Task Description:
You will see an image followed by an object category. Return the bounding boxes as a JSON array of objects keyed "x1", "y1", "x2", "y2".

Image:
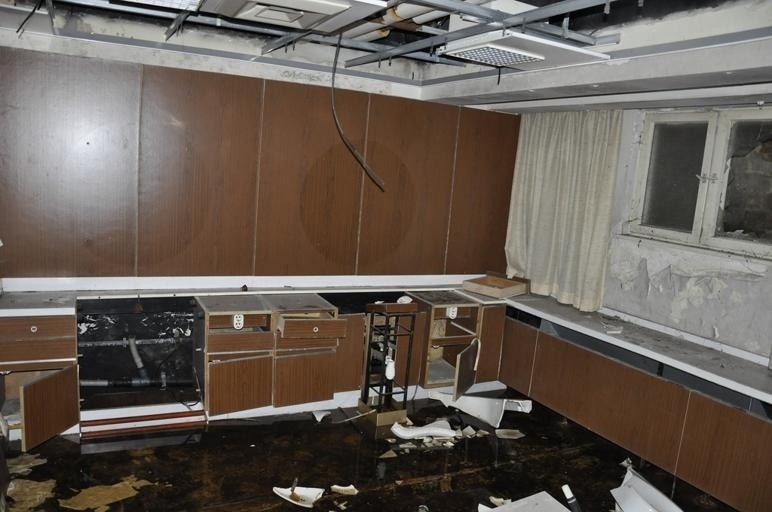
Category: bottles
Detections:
[{"x1": 385, "y1": 355, "x2": 395, "y2": 380}]
